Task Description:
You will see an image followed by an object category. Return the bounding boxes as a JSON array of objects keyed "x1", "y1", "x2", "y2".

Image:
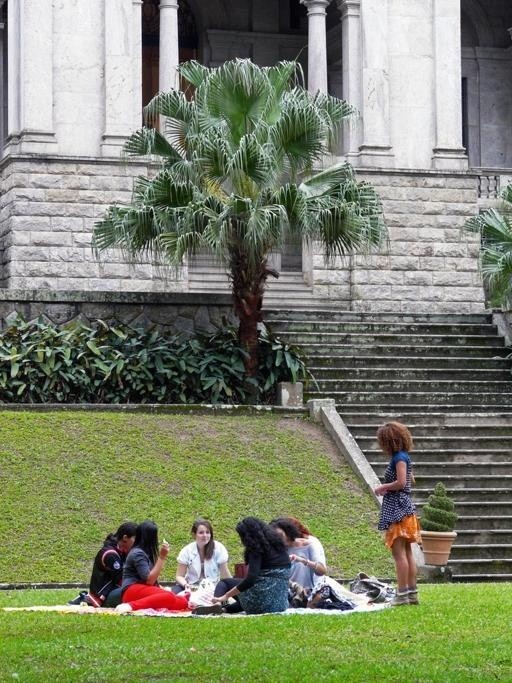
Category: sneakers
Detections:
[
  {"x1": 192, "y1": 604, "x2": 223, "y2": 616},
  {"x1": 387, "y1": 592, "x2": 409, "y2": 607},
  {"x1": 409, "y1": 590, "x2": 419, "y2": 605}
]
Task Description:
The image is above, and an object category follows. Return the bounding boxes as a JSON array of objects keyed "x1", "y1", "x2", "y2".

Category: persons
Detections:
[
  {"x1": 374, "y1": 420, "x2": 422, "y2": 606},
  {"x1": 191, "y1": 516, "x2": 291, "y2": 615},
  {"x1": 113, "y1": 519, "x2": 189, "y2": 614},
  {"x1": 86, "y1": 521, "x2": 139, "y2": 608},
  {"x1": 172, "y1": 519, "x2": 232, "y2": 593},
  {"x1": 269, "y1": 518, "x2": 327, "y2": 608}
]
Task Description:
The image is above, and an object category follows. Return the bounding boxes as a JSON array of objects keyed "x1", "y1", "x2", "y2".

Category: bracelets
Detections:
[{"x1": 313, "y1": 561, "x2": 319, "y2": 572}]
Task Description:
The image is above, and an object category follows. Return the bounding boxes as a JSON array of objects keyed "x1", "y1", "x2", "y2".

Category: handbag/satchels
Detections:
[{"x1": 350, "y1": 572, "x2": 395, "y2": 604}]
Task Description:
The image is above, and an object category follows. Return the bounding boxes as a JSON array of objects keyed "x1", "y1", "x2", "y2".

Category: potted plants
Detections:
[{"x1": 417, "y1": 480, "x2": 459, "y2": 566}]
